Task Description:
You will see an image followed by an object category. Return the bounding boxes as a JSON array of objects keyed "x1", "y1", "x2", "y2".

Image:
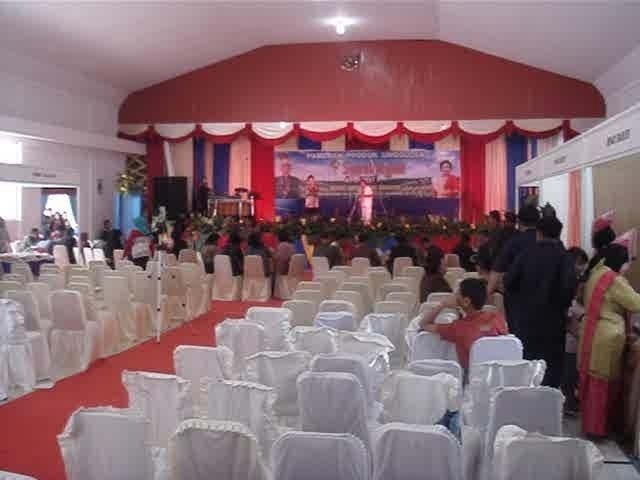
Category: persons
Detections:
[
  {"x1": 0, "y1": 207, "x2": 198, "y2": 273},
  {"x1": 357, "y1": 180, "x2": 374, "y2": 226},
  {"x1": 205, "y1": 213, "x2": 386, "y2": 277},
  {"x1": 304, "y1": 174, "x2": 320, "y2": 223},
  {"x1": 199, "y1": 175, "x2": 209, "y2": 217},
  {"x1": 432, "y1": 160, "x2": 460, "y2": 198},
  {"x1": 277, "y1": 161, "x2": 302, "y2": 199},
  {"x1": 390, "y1": 206, "x2": 639, "y2": 444}
]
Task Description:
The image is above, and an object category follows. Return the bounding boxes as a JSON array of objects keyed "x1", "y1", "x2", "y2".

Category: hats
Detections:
[{"x1": 132, "y1": 216, "x2": 147, "y2": 233}]
